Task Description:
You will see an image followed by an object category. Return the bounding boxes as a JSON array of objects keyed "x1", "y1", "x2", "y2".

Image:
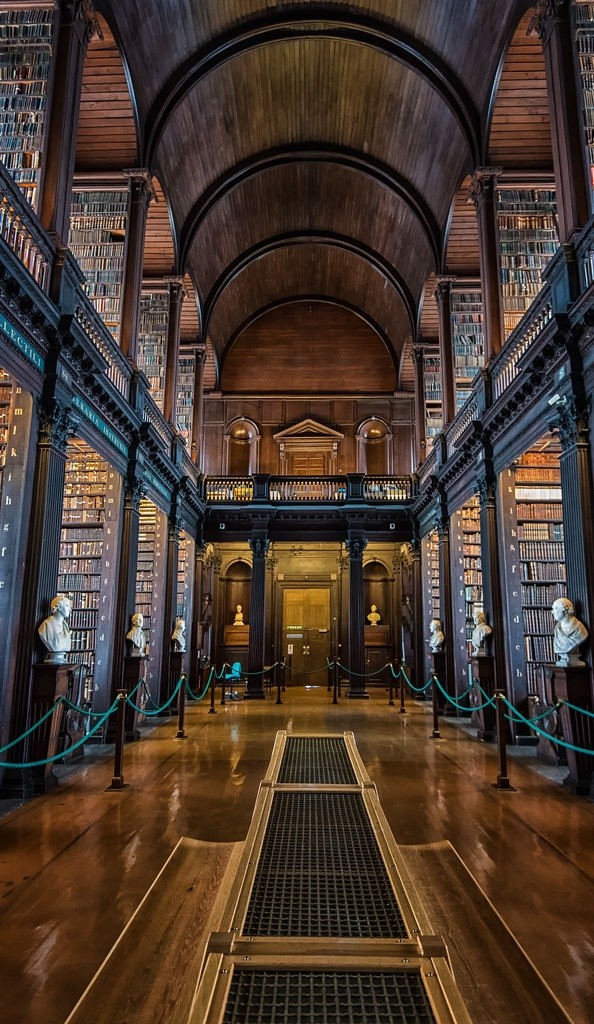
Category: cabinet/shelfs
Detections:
[
  {"x1": 410, "y1": 0, "x2": 594, "y2": 796},
  {"x1": 0, "y1": 0, "x2": 200, "y2": 744}
]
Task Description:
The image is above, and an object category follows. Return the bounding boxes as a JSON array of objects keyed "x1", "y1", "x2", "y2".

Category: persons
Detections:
[
  {"x1": 126, "y1": 614, "x2": 146, "y2": 653},
  {"x1": 171, "y1": 621, "x2": 187, "y2": 651},
  {"x1": 428, "y1": 620, "x2": 444, "y2": 646},
  {"x1": 470, "y1": 611, "x2": 491, "y2": 648},
  {"x1": 367, "y1": 605, "x2": 381, "y2": 621},
  {"x1": 550, "y1": 598, "x2": 588, "y2": 655},
  {"x1": 234, "y1": 604, "x2": 243, "y2": 620},
  {"x1": 38, "y1": 596, "x2": 74, "y2": 652}
]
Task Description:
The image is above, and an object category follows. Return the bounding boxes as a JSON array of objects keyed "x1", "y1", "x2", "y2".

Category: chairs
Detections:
[{"x1": 221, "y1": 661, "x2": 241, "y2": 701}]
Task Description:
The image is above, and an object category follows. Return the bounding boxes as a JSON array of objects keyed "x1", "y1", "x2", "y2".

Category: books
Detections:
[
  {"x1": 56, "y1": 448, "x2": 107, "y2": 705},
  {"x1": 137, "y1": 291, "x2": 168, "y2": 416},
  {"x1": 68, "y1": 190, "x2": 129, "y2": 347},
  {"x1": 498, "y1": 188, "x2": 559, "y2": 345},
  {"x1": 461, "y1": 497, "x2": 484, "y2": 650},
  {"x1": 0, "y1": 7, "x2": 54, "y2": 211},
  {"x1": 175, "y1": 357, "x2": 195, "y2": 456},
  {"x1": 513, "y1": 452, "x2": 567, "y2": 696},
  {"x1": 177, "y1": 529, "x2": 186, "y2": 618},
  {"x1": 134, "y1": 524, "x2": 155, "y2": 656},
  {"x1": 425, "y1": 358, "x2": 442, "y2": 400},
  {"x1": 0, "y1": 368, "x2": 14, "y2": 470},
  {"x1": 430, "y1": 527, "x2": 440, "y2": 619},
  {"x1": 451, "y1": 292, "x2": 483, "y2": 415},
  {"x1": 571, "y1": 0, "x2": 594, "y2": 168}
]
